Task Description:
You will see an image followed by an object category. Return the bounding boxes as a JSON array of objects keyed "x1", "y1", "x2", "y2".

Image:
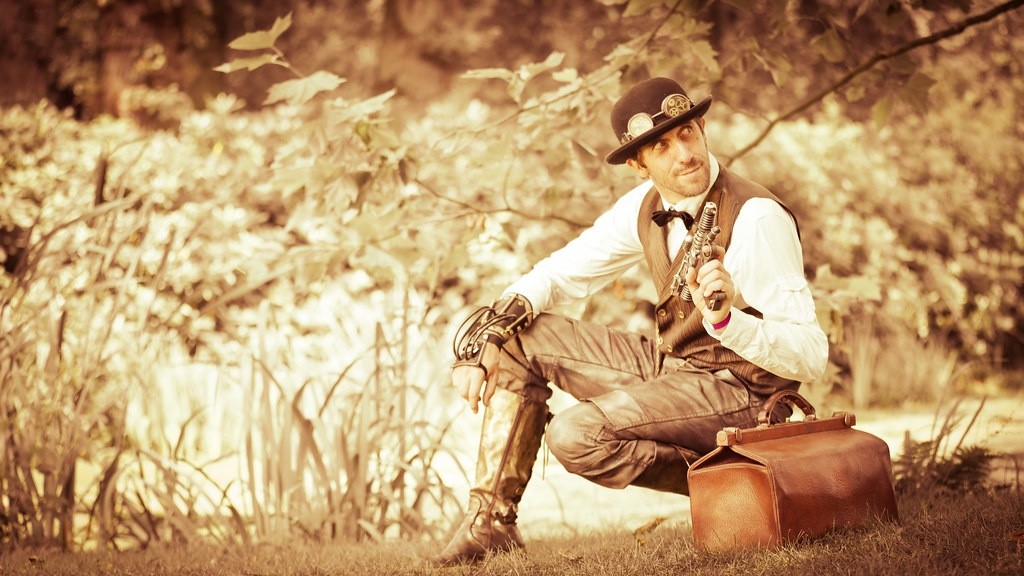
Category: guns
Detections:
[{"x1": 670, "y1": 201, "x2": 725, "y2": 312}]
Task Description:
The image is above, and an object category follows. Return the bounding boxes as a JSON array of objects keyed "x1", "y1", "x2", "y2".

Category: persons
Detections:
[{"x1": 404, "y1": 76, "x2": 830, "y2": 570}]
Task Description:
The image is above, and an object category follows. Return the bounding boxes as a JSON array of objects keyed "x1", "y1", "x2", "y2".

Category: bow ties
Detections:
[{"x1": 651, "y1": 209, "x2": 695, "y2": 231}]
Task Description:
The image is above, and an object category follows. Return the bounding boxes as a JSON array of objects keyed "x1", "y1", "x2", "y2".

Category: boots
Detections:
[
  {"x1": 629, "y1": 439, "x2": 704, "y2": 498},
  {"x1": 418, "y1": 384, "x2": 554, "y2": 568}
]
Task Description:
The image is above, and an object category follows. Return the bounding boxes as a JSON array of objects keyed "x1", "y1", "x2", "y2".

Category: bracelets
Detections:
[{"x1": 710, "y1": 311, "x2": 733, "y2": 331}]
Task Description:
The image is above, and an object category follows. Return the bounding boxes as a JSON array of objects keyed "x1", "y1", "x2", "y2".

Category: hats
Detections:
[{"x1": 605, "y1": 77, "x2": 712, "y2": 165}]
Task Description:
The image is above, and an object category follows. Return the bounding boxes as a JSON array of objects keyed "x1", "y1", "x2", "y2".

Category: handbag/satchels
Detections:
[{"x1": 687, "y1": 389, "x2": 898, "y2": 559}]
